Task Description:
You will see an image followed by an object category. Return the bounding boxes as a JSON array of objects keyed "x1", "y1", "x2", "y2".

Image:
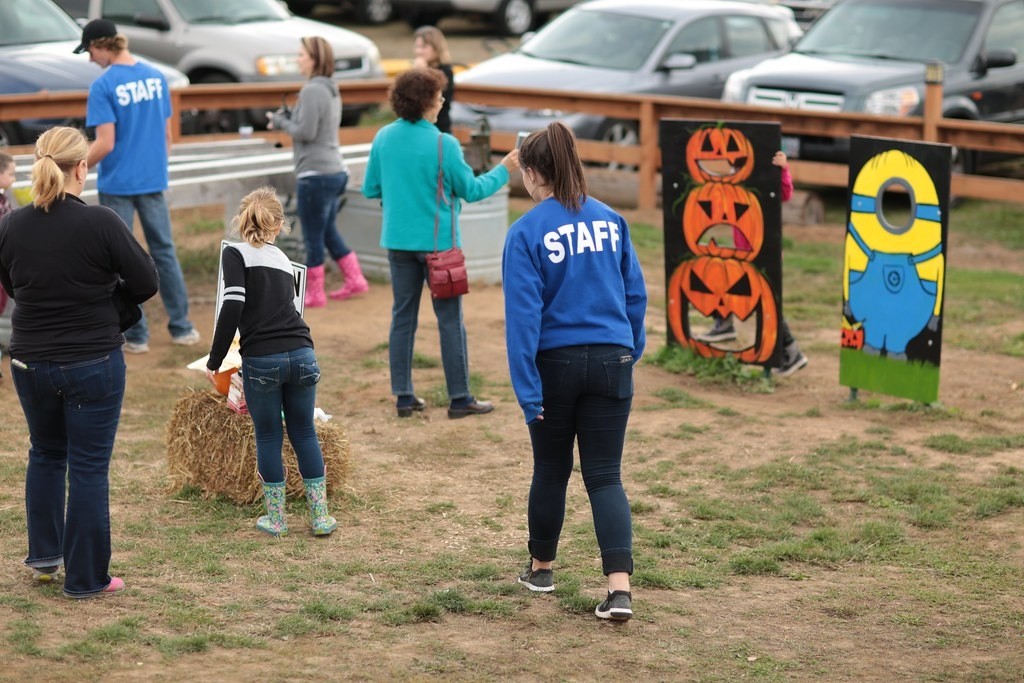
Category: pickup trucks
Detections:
[{"x1": 55, "y1": 0, "x2": 386, "y2": 135}]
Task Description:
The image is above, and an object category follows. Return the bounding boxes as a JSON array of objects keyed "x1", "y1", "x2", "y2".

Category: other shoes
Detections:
[
  {"x1": 448, "y1": 394, "x2": 495, "y2": 418},
  {"x1": 397, "y1": 392, "x2": 425, "y2": 416},
  {"x1": 63, "y1": 577, "x2": 125, "y2": 599},
  {"x1": 122, "y1": 340, "x2": 148, "y2": 354},
  {"x1": 33, "y1": 563, "x2": 58, "y2": 581},
  {"x1": 171, "y1": 328, "x2": 199, "y2": 346}
]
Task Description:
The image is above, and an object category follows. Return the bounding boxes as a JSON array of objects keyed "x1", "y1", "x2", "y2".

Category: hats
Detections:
[{"x1": 74, "y1": 17, "x2": 117, "y2": 55}]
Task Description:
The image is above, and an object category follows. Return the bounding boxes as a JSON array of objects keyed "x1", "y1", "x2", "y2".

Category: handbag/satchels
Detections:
[{"x1": 425, "y1": 247, "x2": 468, "y2": 298}]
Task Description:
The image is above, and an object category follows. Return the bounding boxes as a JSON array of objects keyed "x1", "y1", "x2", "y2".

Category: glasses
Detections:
[{"x1": 427, "y1": 96, "x2": 446, "y2": 104}]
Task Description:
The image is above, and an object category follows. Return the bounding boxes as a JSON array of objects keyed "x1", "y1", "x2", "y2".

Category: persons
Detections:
[
  {"x1": 0, "y1": 127, "x2": 159, "y2": 599},
  {"x1": 502, "y1": 121, "x2": 648, "y2": 621},
  {"x1": 413, "y1": 27, "x2": 454, "y2": 136},
  {"x1": 265, "y1": 36, "x2": 369, "y2": 308},
  {"x1": 72, "y1": 20, "x2": 201, "y2": 353},
  {"x1": 360, "y1": 70, "x2": 521, "y2": 420},
  {"x1": 0, "y1": 153, "x2": 16, "y2": 377},
  {"x1": 206, "y1": 186, "x2": 338, "y2": 537}
]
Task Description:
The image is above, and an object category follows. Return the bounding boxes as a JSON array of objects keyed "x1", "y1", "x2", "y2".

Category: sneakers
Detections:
[
  {"x1": 595, "y1": 589, "x2": 633, "y2": 622},
  {"x1": 776, "y1": 342, "x2": 808, "y2": 377},
  {"x1": 697, "y1": 324, "x2": 737, "y2": 342},
  {"x1": 519, "y1": 557, "x2": 556, "y2": 592}
]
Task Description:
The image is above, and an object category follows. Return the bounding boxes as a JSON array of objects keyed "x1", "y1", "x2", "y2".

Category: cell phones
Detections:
[{"x1": 516, "y1": 132, "x2": 531, "y2": 149}]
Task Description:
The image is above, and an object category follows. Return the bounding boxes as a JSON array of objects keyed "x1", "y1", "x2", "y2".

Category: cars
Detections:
[
  {"x1": 0, "y1": 0, "x2": 203, "y2": 148},
  {"x1": 280, "y1": 0, "x2": 542, "y2": 40},
  {"x1": 718, "y1": 0, "x2": 1024, "y2": 210},
  {"x1": 447, "y1": 0, "x2": 807, "y2": 175}
]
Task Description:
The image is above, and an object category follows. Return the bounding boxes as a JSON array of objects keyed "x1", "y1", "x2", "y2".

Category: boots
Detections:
[
  {"x1": 296, "y1": 465, "x2": 336, "y2": 535},
  {"x1": 255, "y1": 469, "x2": 289, "y2": 536},
  {"x1": 304, "y1": 266, "x2": 327, "y2": 306},
  {"x1": 329, "y1": 250, "x2": 368, "y2": 300}
]
